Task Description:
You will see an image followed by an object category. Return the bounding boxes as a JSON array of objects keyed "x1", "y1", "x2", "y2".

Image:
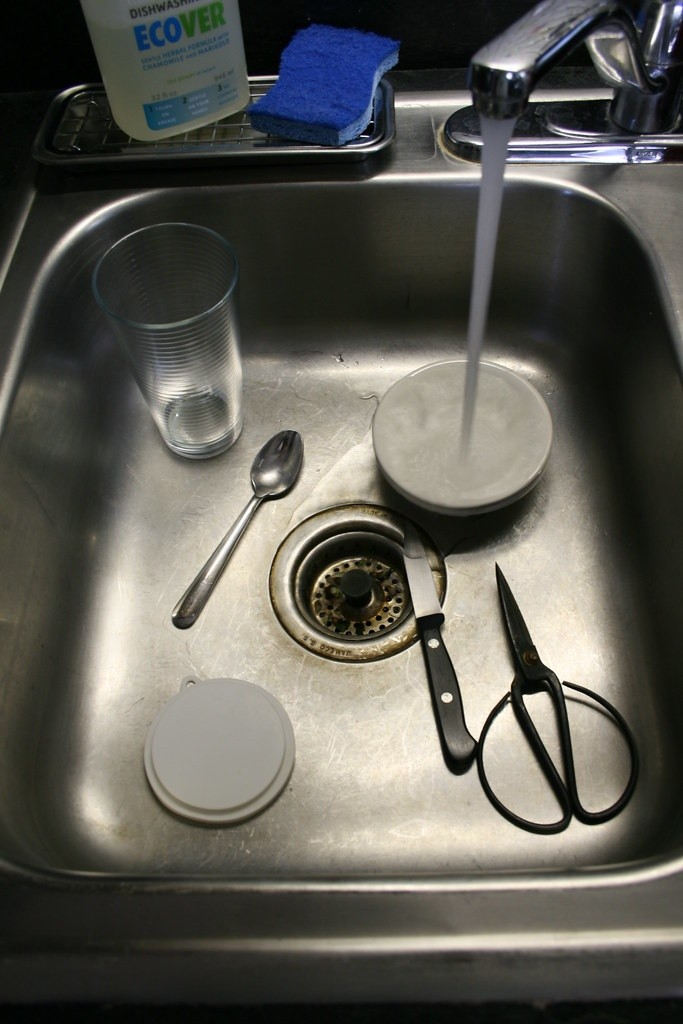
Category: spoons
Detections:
[{"x1": 171, "y1": 430, "x2": 304, "y2": 630}]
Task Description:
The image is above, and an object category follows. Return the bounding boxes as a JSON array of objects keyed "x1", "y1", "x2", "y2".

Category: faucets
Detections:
[{"x1": 467, "y1": 0, "x2": 683, "y2": 137}]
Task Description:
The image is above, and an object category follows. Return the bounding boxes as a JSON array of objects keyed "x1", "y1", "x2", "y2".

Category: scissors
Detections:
[{"x1": 477, "y1": 560, "x2": 641, "y2": 837}]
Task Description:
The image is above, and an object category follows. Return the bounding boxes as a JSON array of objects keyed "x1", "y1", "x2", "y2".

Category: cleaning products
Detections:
[{"x1": 78, "y1": 1, "x2": 253, "y2": 144}]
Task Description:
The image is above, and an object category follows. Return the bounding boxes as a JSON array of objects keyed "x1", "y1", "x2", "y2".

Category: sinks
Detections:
[{"x1": 0, "y1": 74, "x2": 683, "y2": 1009}]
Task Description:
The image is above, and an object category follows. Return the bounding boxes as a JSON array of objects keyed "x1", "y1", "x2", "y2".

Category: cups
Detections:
[{"x1": 91, "y1": 221, "x2": 244, "y2": 460}]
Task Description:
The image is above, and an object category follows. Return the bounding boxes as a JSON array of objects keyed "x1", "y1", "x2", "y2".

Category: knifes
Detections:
[{"x1": 402, "y1": 519, "x2": 478, "y2": 776}]
